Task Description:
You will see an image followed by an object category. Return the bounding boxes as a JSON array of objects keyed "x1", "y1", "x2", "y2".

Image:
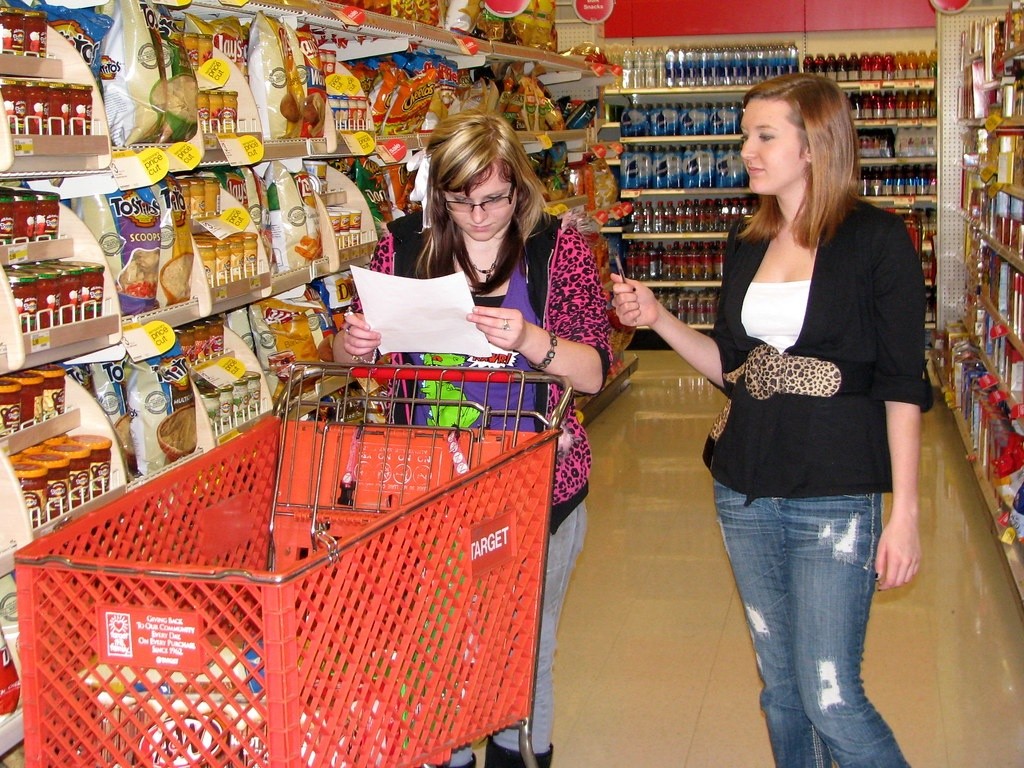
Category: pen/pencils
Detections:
[{"x1": 613, "y1": 250, "x2": 628, "y2": 285}]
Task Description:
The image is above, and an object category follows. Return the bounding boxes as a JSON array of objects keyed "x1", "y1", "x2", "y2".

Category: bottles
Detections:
[
  {"x1": 604, "y1": 41, "x2": 801, "y2": 331},
  {"x1": 803, "y1": 49, "x2": 940, "y2": 199}
]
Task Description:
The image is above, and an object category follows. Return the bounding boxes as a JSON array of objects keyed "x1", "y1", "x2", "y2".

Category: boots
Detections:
[{"x1": 484, "y1": 736, "x2": 554, "y2": 768}]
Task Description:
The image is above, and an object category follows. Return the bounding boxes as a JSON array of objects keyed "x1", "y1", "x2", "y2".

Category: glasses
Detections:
[{"x1": 442, "y1": 182, "x2": 516, "y2": 213}]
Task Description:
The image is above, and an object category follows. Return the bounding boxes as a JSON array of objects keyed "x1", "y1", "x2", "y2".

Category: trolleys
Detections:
[{"x1": 12, "y1": 357, "x2": 573, "y2": 768}]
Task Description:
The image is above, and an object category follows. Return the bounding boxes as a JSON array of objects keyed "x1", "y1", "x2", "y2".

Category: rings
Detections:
[
  {"x1": 346, "y1": 326, "x2": 351, "y2": 334},
  {"x1": 503, "y1": 320, "x2": 508, "y2": 330}
]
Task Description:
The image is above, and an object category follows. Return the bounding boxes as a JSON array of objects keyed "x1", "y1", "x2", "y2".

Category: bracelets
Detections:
[
  {"x1": 527, "y1": 332, "x2": 557, "y2": 370},
  {"x1": 356, "y1": 349, "x2": 377, "y2": 364}
]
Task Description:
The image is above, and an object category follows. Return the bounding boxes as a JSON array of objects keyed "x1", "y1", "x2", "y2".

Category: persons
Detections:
[
  {"x1": 334, "y1": 109, "x2": 613, "y2": 768},
  {"x1": 610, "y1": 73, "x2": 934, "y2": 768}
]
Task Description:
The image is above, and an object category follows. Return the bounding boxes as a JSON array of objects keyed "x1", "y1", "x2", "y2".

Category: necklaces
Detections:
[{"x1": 469, "y1": 256, "x2": 496, "y2": 281}]
